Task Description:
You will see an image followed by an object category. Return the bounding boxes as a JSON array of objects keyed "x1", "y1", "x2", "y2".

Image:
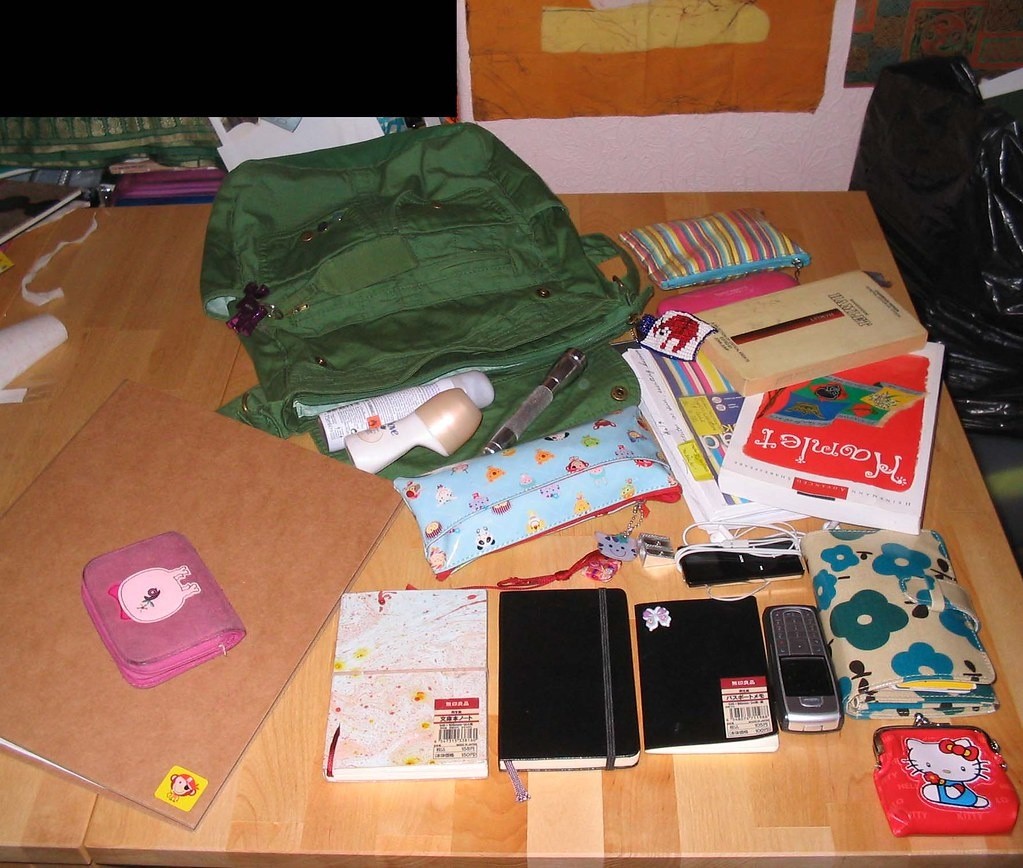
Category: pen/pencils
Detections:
[{"x1": 479, "y1": 347, "x2": 589, "y2": 457}]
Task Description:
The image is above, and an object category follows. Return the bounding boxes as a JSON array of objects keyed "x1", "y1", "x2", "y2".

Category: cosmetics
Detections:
[{"x1": 319, "y1": 370, "x2": 494, "y2": 474}]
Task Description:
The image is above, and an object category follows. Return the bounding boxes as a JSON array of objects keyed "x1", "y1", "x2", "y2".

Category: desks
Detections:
[{"x1": 0, "y1": 193, "x2": 1023, "y2": 868}]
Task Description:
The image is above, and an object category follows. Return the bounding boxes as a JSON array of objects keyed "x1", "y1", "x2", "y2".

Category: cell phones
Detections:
[
  {"x1": 677, "y1": 537, "x2": 804, "y2": 588},
  {"x1": 762, "y1": 605, "x2": 844, "y2": 735}
]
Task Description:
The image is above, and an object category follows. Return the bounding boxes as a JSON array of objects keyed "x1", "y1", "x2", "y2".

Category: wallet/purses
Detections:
[
  {"x1": 802, "y1": 528, "x2": 1001, "y2": 719},
  {"x1": 79, "y1": 530, "x2": 247, "y2": 688},
  {"x1": 873, "y1": 713, "x2": 1020, "y2": 837}
]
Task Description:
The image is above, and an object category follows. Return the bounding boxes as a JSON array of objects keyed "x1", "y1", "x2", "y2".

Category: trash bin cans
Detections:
[{"x1": 849, "y1": 53, "x2": 1023, "y2": 579}]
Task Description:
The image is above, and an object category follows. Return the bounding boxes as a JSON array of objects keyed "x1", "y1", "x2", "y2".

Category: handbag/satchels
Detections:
[
  {"x1": 620, "y1": 208, "x2": 811, "y2": 287},
  {"x1": 394, "y1": 405, "x2": 681, "y2": 580}
]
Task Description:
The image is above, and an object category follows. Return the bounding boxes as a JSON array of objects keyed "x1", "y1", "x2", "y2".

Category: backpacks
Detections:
[{"x1": 198, "y1": 121, "x2": 654, "y2": 481}]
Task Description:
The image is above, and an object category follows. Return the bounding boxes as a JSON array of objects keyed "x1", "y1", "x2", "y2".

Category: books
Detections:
[
  {"x1": 717, "y1": 342, "x2": 945, "y2": 535},
  {"x1": 498, "y1": 588, "x2": 641, "y2": 772},
  {"x1": 693, "y1": 270, "x2": 928, "y2": 397},
  {"x1": 0, "y1": 180, "x2": 81, "y2": 247},
  {"x1": 0, "y1": 380, "x2": 406, "y2": 831},
  {"x1": 635, "y1": 596, "x2": 781, "y2": 755},
  {"x1": 322, "y1": 588, "x2": 489, "y2": 783}
]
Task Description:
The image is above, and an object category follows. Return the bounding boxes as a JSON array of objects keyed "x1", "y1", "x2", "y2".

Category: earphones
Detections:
[{"x1": 710, "y1": 532, "x2": 726, "y2": 543}]
